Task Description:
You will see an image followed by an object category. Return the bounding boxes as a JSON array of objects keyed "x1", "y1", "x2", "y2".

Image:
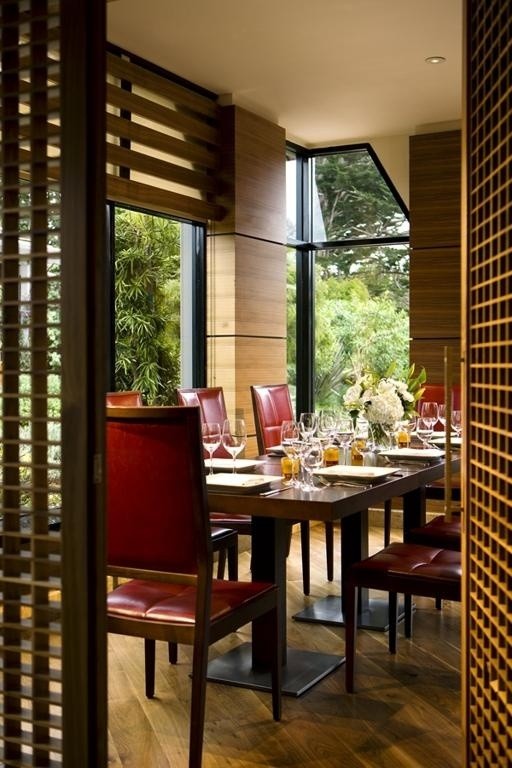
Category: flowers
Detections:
[{"x1": 343, "y1": 359, "x2": 426, "y2": 443}]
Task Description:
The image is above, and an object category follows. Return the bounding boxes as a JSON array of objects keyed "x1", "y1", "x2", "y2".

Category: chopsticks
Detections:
[{"x1": 204, "y1": 457, "x2": 268, "y2": 471}]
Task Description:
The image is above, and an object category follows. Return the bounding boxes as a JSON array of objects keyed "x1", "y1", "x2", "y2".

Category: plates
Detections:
[
  {"x1": 205, "y1": 471, "x2": 286, "y2": 493},
  {"x1": 377, "y1": 448, "x2": 446, "y2": 460},
  {"x1": 265, "y1": 444, "x2": 287, "y2": 454},
  {"x1": 407, "y1": 430, "x2": 456, "y2": 438},
  {"x1": 427, "y1": 436, "x2": 463, "y2": 444},
  {"x1": 313, "y1": 464, "x2": 402, "y2": 482}
]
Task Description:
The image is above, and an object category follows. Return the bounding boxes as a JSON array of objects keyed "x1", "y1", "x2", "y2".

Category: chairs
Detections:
[
  {"x1": 107, "y1": 406, "x2": 281, "y2": 767},
  {"x1": 346, "y1": 542, "x2": 461, "y2": 693},
  {"x1": 385, "y1": 384, "x2": 461, "y2": 547},
  {"x1": 107, "y1": 391, "x2": 238, "y2": 665},
  {"x1": 404, "y1": 516, "x2": 461, "y2": 637},
  {"x1": 251, "y1": 385, "x2": 334, "y2": 596},
  {"x1": 177, "y1": 388, "x2": 309, "y2": 595}
]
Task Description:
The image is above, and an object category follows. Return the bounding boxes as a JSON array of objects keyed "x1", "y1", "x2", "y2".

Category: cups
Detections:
[{"x1": 438, "y1": 404, "x2": 446, "y2": 425}]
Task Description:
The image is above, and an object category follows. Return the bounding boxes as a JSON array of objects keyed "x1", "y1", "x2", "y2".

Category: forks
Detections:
[{"x1": 317, "y1": 475, "x2": 368, "y2": 490}]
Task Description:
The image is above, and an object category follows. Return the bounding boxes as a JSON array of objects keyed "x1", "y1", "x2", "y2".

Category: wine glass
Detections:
[
  {"x1": 221, "y1": 418, "x2": 247, "y2": 474},
  {"x1": 416, "y1": 417, "x2": 434, "y2": 449},
  {"x1": 450, "y1": 410, "x2": 461, "y2": 438},
  {"x1": 421, "y1": 401, "x2": 439, "y2": 430},
  {"x1": 201, "y1": 422, "x2": 223, "y2": 475},
  {"x1": 281, "y1": 410, "x2": 369, "y2": 494}
]
